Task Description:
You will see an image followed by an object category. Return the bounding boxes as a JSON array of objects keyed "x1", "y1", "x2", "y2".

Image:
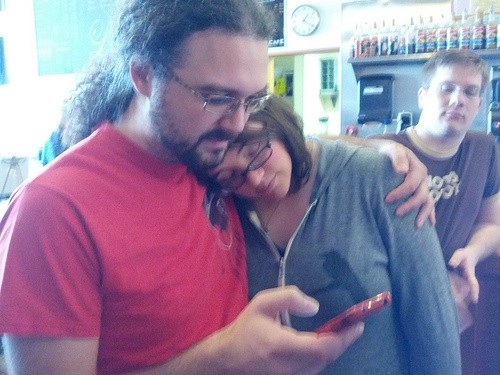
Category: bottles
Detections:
[
  {"x1": 316, "y1": 115, "x2": 329, "y2": 140},
  {"x1": 349, "y1": 0, "x2": 500, "y2": 57}
]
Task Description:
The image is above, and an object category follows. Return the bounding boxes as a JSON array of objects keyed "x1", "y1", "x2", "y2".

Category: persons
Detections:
[
  {"x1": 207, "y1": 92, "x2": 461, "y2": 375},
  {"x1": 370, "y1": 48, "x2": 500, "y2": 304},
  {"x1": 0, "y1": 20, "x2": 436, "y2": 375}
]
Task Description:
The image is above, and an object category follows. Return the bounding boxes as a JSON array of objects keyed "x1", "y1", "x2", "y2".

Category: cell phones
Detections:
[{"x1": 312, "y1": 291, "x2": 393, "y2": 335}]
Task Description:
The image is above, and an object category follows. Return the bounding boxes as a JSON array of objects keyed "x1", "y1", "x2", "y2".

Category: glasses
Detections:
[
  {"x1": 216, "y1": 122, "x2": 273, "y2": 197},
  {"x1": 162, "y1": 69, "x2": 272, "y2": 116}
]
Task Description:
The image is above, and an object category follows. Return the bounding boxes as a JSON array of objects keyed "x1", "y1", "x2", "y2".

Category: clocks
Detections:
[{"x1": 289, "y1": 4, "x2": 321, "y2": 36}]
